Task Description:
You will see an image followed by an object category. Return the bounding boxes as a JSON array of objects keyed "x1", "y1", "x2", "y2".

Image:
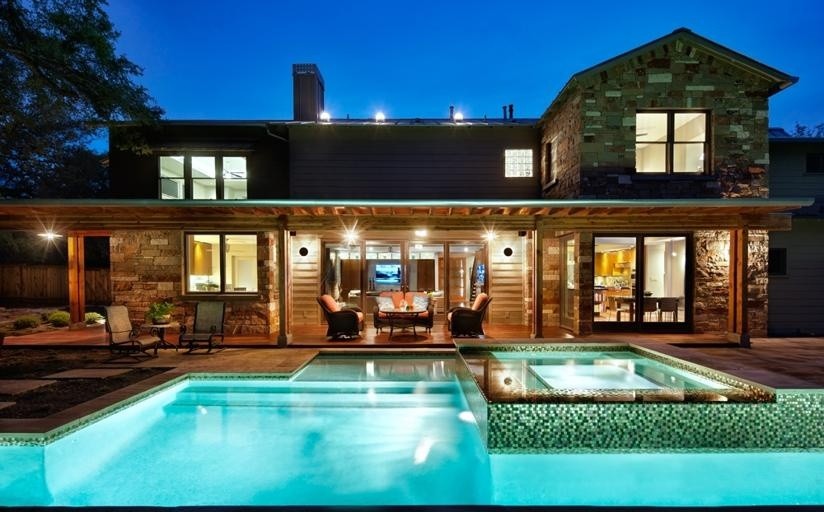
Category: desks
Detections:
[
  {"x1": 381, "y1": 308, "x2": 425, "y2": 340},
  {"x1": 141, "y1": 321, "x2": 181, "y2": 355}
]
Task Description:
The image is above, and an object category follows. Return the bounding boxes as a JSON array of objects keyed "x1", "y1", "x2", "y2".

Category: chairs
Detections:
[
  {"x1": 447, "y1": 292, "x2": 493, "y2": 338},
  {"x1": 103, "y1": 305, "x2": 161, "y2": 362},
  {"x1": 608, "y1": 296, "x2": 684, "y2": 322},
  {"x1": 179, "y1": 302, "x2": 227, "y2": 355},
  {"x1": 316, "y1": 294, "x2": 365, "y2": 339}
]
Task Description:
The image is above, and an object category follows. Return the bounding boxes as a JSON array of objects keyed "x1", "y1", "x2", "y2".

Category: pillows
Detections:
[
  {"x1": 376, "y1": 296, "x2": 394, "y2": 312},
  {"x1": 413, "y1": 296, "x2": 428, "y2": 311}
]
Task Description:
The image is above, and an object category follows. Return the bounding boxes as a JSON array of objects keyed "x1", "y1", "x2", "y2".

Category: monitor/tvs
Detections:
[
  {"x1": 469, "y1": 264, "x2": 486, "y2": 286},
  {"x1": 373, "y1": 262, "x2": 402, "y2": 286}
]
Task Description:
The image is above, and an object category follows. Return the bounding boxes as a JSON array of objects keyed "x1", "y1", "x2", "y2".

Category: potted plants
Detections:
[{"x1": 146, "y1": 301, "x2": 172, "y2": 323}]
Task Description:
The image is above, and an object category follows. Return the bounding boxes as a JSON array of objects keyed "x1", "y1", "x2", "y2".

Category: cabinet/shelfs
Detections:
[{"x1": 594, "y1": 252, "x2": 632, "y2": 287}]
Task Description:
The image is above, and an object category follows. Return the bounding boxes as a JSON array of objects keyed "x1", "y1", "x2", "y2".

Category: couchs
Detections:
[{"x1": 375, "y1": 291, "x2": 433, "y2": 333}]
